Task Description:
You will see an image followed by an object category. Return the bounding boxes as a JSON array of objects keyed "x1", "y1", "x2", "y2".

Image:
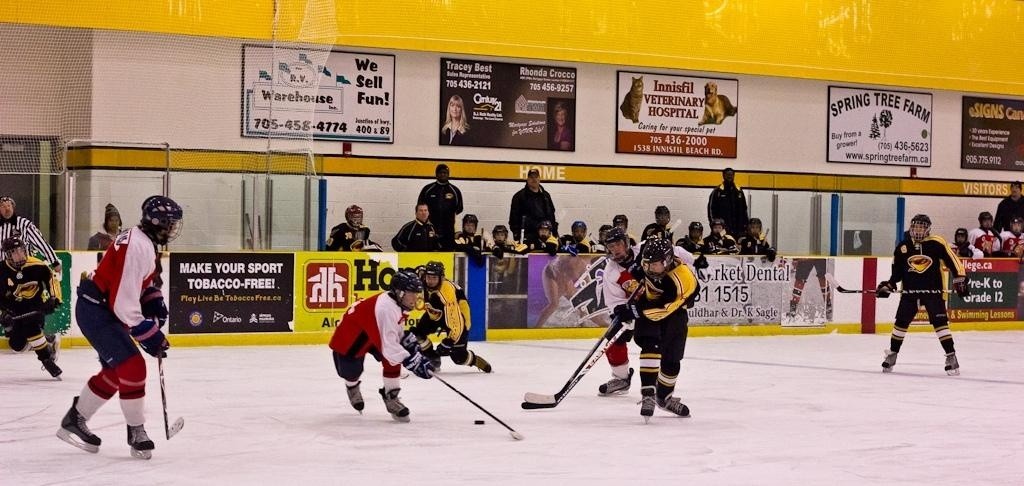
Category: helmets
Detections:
[
  {"x1": 345, "y1": 205, "x2": 363, "y2": 226},
  {"x1": 604, "y1": 226, "x2": 633, "y2": 253},
  {"x1": 1, "y1": 237, "x2": 28, "y2": 269},
  {"x1": 142, "y1": 195, "x2": 184, "y2": 244},
  {"x1": 389, "y1": 270, "x2": 423, "y2": 311},
  {"x1": 425, "y1": 260, "x2": 445, "y2": 288},
  {"x1": 613, "y1": 214, "x2": 628, "y2": 233},
  {"x1": 953, "y1": 228, "x2": 968, "y2": 247},
  {"x1": 600, "y1": 224, "x2": 612, "y2": 244},
  {"x1": 978, "y1": 212, "x2": 993, "y2": 229},
  {"x1": 1009, "y1": 216, "x2": 1024, "y2": 235},
  {"x1": 570, "y1": 221, "x2": 587, "y2": 240},
  {"x1": 640, "y1": 238, "x2": 675, "y2": 278},
  {"x1": 747, "y1": 218, "x2": 762, "y2": 238},
  {"x1": 655, "y1": 206, "x2": 670, "y2": 225},
  {"x1": 909, "y1": 214, "x2": 933, "y2": 238},
  {"x1": 493, "y1": 225, "x2": 508, "y2": 240},
  {"x1": 464, "y1": 214, "x2": 478, "y2": 236},
  {"x1": 688, "y1": 221, "x2": 703, "y2": 239},
  {"x1": 538, "y1": 220, "x2": 553, "y2": 239},
  {"x1": 710, "y1": 219, "x2": 724, "y2": 234}
]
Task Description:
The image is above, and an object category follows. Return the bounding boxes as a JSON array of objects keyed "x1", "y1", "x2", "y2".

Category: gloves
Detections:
[
  {"x1": 129, "y1": 320, "x2": 171, "y2": 359},
  {"x1": 38, "y1": 300, "x2": 57, "y2": 315},
  {"x1": 0, "y1": 308, "x2": 17, "y2": 330},
  {"x1": 141, "y1": 286, "x2": 168, "y2": 329},
  {"x1": 400, "y1": 331, "x2": 455, "y2": 379},
  {"x1": 953, "y1": 277, "x2": 971, "y2": 297},
  {"x1": 613, "y1": 305, "x2": 642, "y2": 321},
  {"x1": 876, "y1": 280, "x2": 897, "y2": 297}
]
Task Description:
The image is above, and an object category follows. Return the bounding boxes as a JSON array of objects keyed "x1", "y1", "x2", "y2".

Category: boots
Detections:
[
  {"x1": 944, "y1": 353, "x2": 959, "y2": 376},
  {"x1": 56, "y1": 395, "x2": 101, "y2": 453},
  {"x1": 38, "y1": 333, "x2": 63, "y2": 382},
  {"x1": 466, "y1": 353, "x2": 493, "y2": 373},
  {"x1": 656, "y1": 391, "x2": 691, "y2": 419},
  {"x1": 125, "y1": 423, "x2": 155, "y2": 460},
  {"x1": 882, "y1": 349, "x2": 897, "y2": 373},
  {"x1": 597, "y1": 367, "x2": 633, "y2": 397},
  {"x1": 637, "y1": 385, "x2": 658, "y2": 423},
  {"x1": 346, "y1": 381, "x2": 365, "y2": 415},
  {"x1": 379, "y1": 386, "x2": 409, "y2": 422}
]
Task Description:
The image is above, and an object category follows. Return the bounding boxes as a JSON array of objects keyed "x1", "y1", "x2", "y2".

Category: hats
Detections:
[
  {"x1": 1011, "y1": 180, "x2": 1021, "y2": 190},
  {"x1": 104, "y1": 203, "x2": 123, "y2": 231},
  {"x1": 528, "y1": 168, "x2": 540, "y2": 178},
  {"x1": 723, "y1": 167, "x2": 736, "y2": 174},
  {"x1": 434, "y1": 162, "x2": 450, "y2": 173}
]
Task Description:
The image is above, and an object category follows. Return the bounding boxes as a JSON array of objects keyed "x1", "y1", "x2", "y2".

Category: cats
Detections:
[{"x1": 619, "y1": 75, "x2": 644, "y2": 123}]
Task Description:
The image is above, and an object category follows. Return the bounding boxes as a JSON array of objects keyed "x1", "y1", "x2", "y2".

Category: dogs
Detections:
[{"x1": 697, "y1": 82, "x2": 736, "y2": 126}]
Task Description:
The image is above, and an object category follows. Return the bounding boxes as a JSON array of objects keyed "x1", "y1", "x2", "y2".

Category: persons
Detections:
[
  {"x1": 413, "y1": 164, "x2": 463, "y2": 246},
  {"x1": 998, "y1": 212, "x2": 1023, "y2": 258},
  {"x1": 994, "y1": 180, "x2": 1023, "y2": 233},
  {"x1": 55, "y1": 194, "x2": 183, "y2": 461},
  {"x1": 596, "y1": 227, "x2": 710, "y2": 396},
  {"x1": 613, "y1": 236, "x2": 691, "y2": 425},
  {"x1": 88, "y1": 203, "x2": 125, "y2": 250},
  {"x1": 967, "y1": 211, "x2": 1002, "y2": 257},
  {"x1": 595, "y1": 206, "x2": 776, "y2": 262},
  {"x1": 453, "y1": 214, "x2": 597, "y2": 259},
  {"x1": 0, "y1": 239, "x2": 65, "y2": 384},
  {"x1": 390, "y1": 199, "x2": 445, "y2": 251},
  {"x1": 438, "y1": 94, "x2": 476, "y2": 146},
  {"x1": 329, "y1": 270, "x2": 435, "y2": 422},
  {"x1": 947, "y1": 226, "x2": 983, "y2": 259},
  {"x1": 0, "y1": 197, "x2": 63, "y2": 275},
  {"x1": 708, "y1": 168, "x2": 748, "y2": 233},
  {"x1": 325, "y1": 204, "x2": 381, "y2": 250},
  {"x1": 547, "y1": 101, "x2": 574, "y2": 150},
  {"x1": 507, "y1": 169, "x2": 556, "y2": 243},
  {"x1": 411, "y1": 259, "x2": 496, "y2": 375},
  {"x1": 873, "y1": 213, "x2": 967, "y2": 375}
]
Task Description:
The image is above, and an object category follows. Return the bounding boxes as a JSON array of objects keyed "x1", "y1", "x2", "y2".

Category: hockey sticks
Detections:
[
  {"x1": 824, "y1": 271, "x2": 957, "y2": 294},
  {"x1": 156, "y1": 348, "x2": 184, "y2": 439},
  {"x1": 426, "y1": 369, "x2": 524, "y2": 440},
  {"x1": 522, "y1": 280, "x2": 646, "y2": 409}
]
